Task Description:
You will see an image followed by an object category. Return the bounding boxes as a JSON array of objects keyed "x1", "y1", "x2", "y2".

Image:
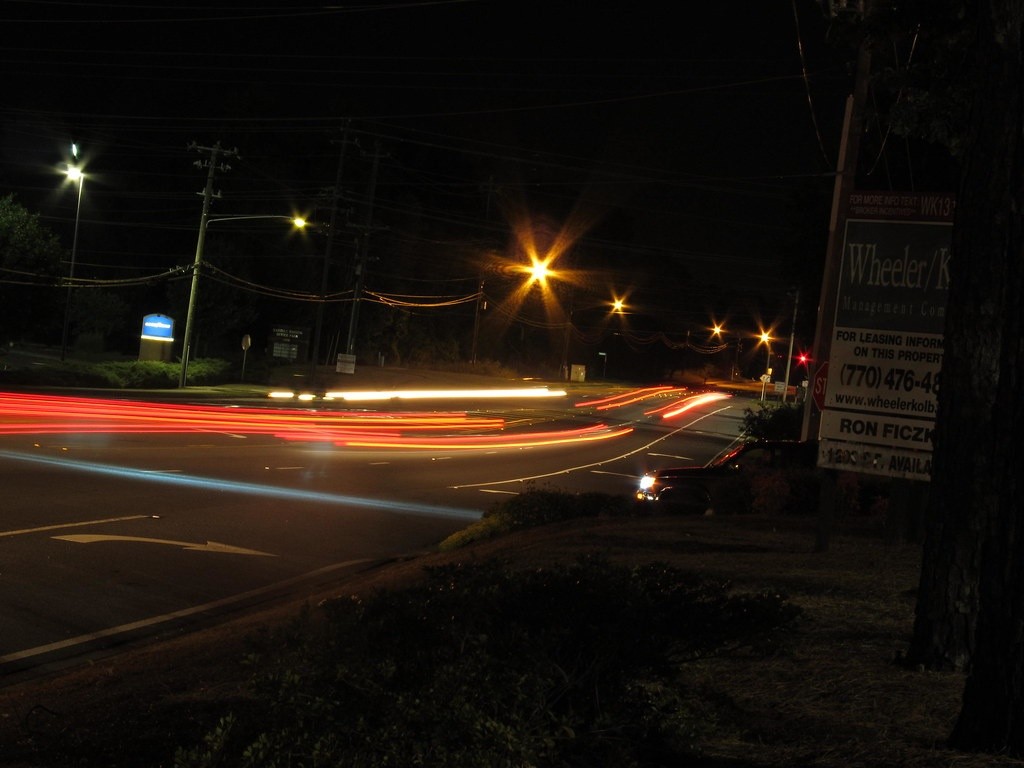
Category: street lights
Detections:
[
  {"x1": 60, "y1": 159, "x2": 93, "y2": 365},
  {"x1": 755, "y1": 330, "x2": 770, "y2": 402},
  {"x1": 470, "y1": 253, "x2": 547, "y2": 365},
  {"x1": 684, "y1": 325, "x2": 720, "y2": 380},
  {"x1": 178, "y1": 143, "x2": 310, "y2": 388},
  {"x1": 798, "y1": 344, "x2": 810, "y2": 379},
  {"x1": 561, "y1": 258, "x2": 624, "y2": 379}
]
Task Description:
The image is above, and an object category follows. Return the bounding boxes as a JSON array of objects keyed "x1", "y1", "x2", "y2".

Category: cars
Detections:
[{"x1": 635, "y1": 437, "x2": 890, "y2": 527}]
